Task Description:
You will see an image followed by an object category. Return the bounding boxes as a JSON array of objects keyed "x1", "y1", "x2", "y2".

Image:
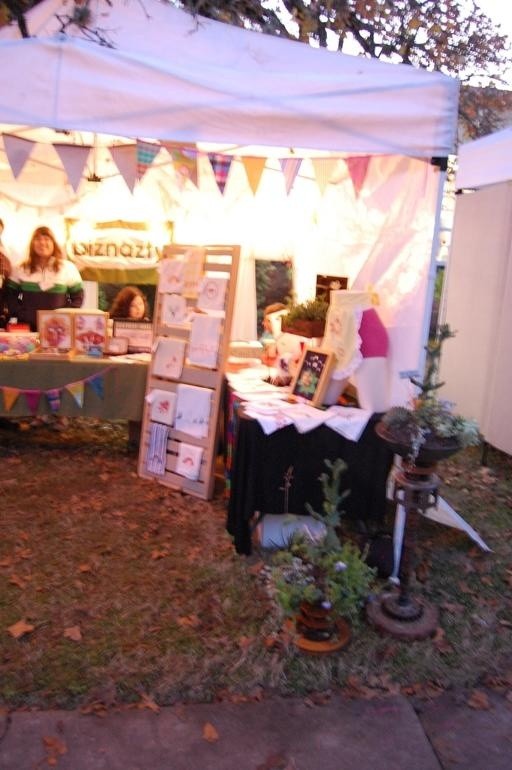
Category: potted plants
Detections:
[
  {"x1": 282, "y1": 292, "x2": 328, "y2": 338},
  {"x1": 258, "y1": 460, "x2": 377, "y2": 652}
]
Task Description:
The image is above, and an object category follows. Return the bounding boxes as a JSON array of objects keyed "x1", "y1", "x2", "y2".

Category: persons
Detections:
[
  {"x1": 5, "y1": 224, "x2": 85, "y2": 434},
  {"x1": 255, "y1": 301, "x2": 295, "y2": 345},
  {"x1": 0, "y1": 217, "x2": 13, "y2": 292},
  {"x1": 101, "y1": 282, "x2": 154, "y2": 326}
]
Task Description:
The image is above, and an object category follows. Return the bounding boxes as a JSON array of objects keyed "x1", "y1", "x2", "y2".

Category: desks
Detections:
[
  {"x1": 0, "y1": 334, "x2": 150, "y2": 448},
  {"x1": 221, "y1": 342, "x2": 394, "y2": 557}
]
